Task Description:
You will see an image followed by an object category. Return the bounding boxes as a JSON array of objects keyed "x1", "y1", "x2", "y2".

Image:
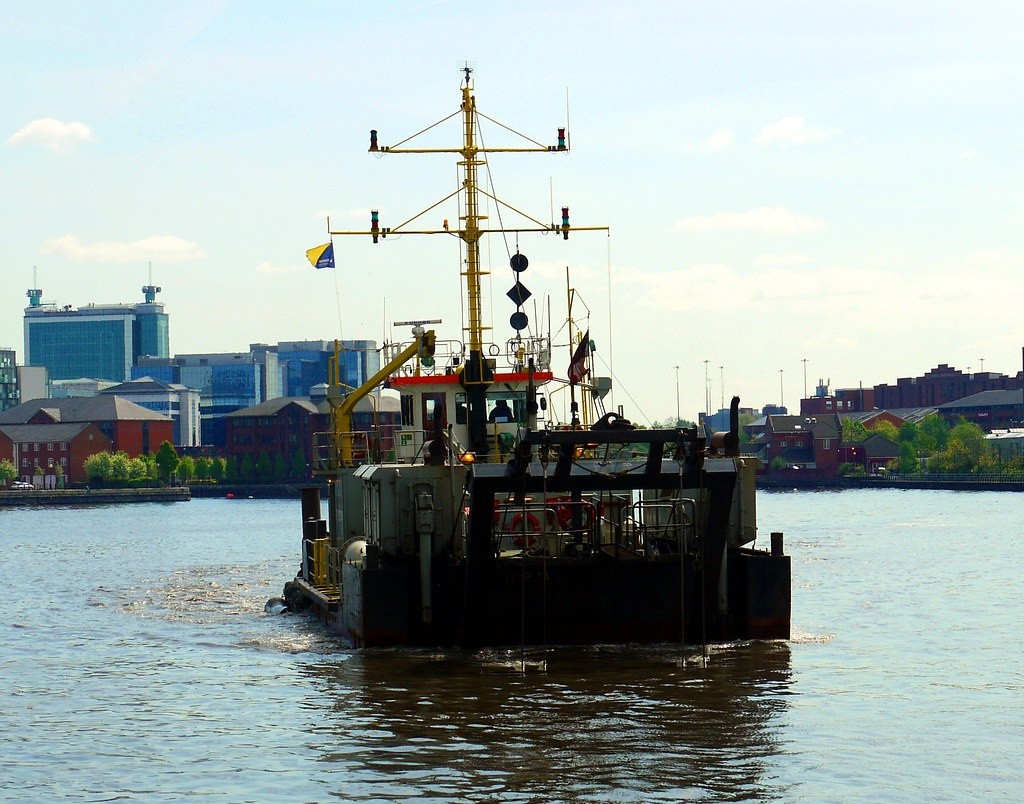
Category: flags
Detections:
[{"x1": 306, "y1": 241, "x2": 335, "y2": 269}]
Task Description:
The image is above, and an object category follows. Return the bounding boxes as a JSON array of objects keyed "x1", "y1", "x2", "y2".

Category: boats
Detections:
[{"x1": 279, "y1": 63, "x2": 795, "y2": 643}]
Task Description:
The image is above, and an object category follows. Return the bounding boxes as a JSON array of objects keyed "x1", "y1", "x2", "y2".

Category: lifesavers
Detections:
[
  {"x1": 562, "y1": 425, "x2": 583, "y2": 430},
  {"x1": 350, "y1": 432, "x2": 373, "y2": 458},
  {"x1": 508, "y1": 512, "x2": 543, "y2": 547},
  {"x1": 558, "y1": 497, "x2": 595, "y2": 532}
]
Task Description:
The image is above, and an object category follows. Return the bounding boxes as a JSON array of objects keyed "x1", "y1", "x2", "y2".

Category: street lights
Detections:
[
  {"x1": 670, "y1": 365, "x2": 680, "y2": 422},
  {"x1": 801, "y1": 358, "x2": 809, "y2": 399},
  {"x1": 701, "y1": 359, "x2": 711, "y2": 416},
  {"x1": 716, "y1": 365, "x2": 724, "y2": 410},
  {"x1": 777, "y1": 369, "x2": 785, "y2": 406}
]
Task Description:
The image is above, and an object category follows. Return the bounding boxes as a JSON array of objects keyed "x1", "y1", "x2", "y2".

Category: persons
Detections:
[{"x1": 489, "y1": 400, "x2": 514, "y2": 423}]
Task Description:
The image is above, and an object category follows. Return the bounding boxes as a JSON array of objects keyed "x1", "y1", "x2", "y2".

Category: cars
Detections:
[{"x1": 11, "y1": 482, "x2": 34, "y2": 491}]
